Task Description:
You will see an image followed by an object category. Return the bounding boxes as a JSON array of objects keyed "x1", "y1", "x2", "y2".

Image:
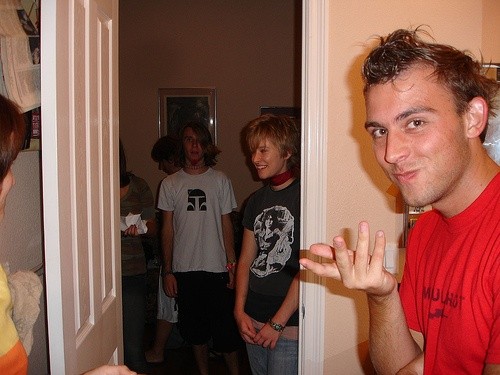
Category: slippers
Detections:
[{"x1": 143, "y1": 352, "x2": 166, "y2": 365}]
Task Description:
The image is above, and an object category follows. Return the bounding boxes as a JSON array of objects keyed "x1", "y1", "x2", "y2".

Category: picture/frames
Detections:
[{"x1": 157, "y1": 87, "x2": 217, "y2": 155}]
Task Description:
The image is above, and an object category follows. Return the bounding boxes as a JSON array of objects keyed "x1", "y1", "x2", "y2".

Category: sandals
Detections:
[{"x1": 208, "y1": 350, "x2": 223, "y2": 360}]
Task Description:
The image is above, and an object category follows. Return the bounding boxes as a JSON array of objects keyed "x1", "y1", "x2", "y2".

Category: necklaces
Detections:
[
  {"x1": 185, "y1": 165, "x2": 206, "y2": 170},
  {"x1": 270, "y1": 170, "x2": 294, "y2": 186}
]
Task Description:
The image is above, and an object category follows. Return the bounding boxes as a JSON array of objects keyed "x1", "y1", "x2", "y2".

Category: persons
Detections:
[
  {"x1": 144, "y1": 135, "x2": 222, "y2": 363},
  {"x1": 157, "y1": 120, "x2": 240, "y2": 375},
  {"x1": 119, "y1": 141, "x2": 157, "y2": 375},
  {"x1": 0, "y1": 93, "x2": 30, "y2": 375},
  {"x1": 299, "y1": 24, "x2": 500, "y2": 375},
  {"x1": 234, "y1": 114, "x2": 301, "y2": 375}
]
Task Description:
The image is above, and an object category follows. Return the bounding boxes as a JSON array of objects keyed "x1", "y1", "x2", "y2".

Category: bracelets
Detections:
[
  {"x1": 225, "y1": 259, "x2": 237, "y2": 271},
  {"x1": 161, "y1": 270, "x2": 172, "y2": 277},
  {"x1": 268, "y1": 319, "x2": 286, "y2": 332}
]
